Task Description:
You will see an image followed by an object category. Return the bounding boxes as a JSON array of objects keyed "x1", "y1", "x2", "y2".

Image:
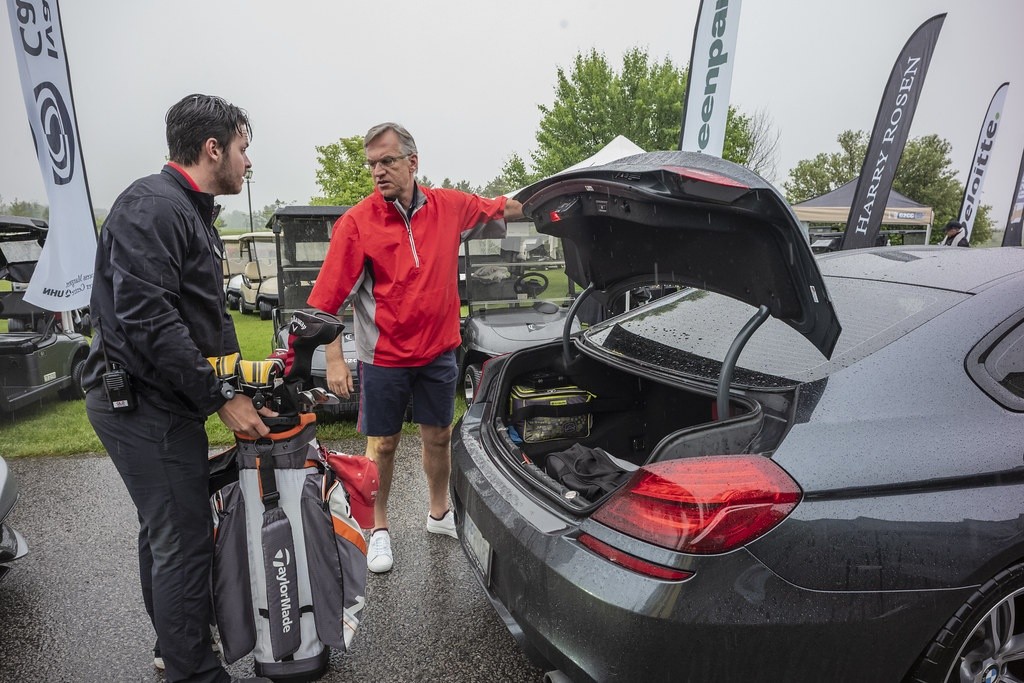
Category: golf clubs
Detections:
[{"x1": 299, "y1": 387, "x2": 341, "y2": 413}]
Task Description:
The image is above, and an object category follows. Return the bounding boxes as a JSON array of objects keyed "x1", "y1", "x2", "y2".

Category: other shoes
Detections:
[
  {"x1": 232, "y1": 676, "x2": 273, "y2": 683},
  {"x1": 155, "y1": 633, "x2": 218, "y2": 669}
]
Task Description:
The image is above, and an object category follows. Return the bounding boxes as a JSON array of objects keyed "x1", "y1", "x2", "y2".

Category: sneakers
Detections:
[
  {"x1": 427, "y1": 510, "x2": 459, "y2": 539},
  {"x1": 367, "y1": 528, "x2": 393, "y2": 573}
]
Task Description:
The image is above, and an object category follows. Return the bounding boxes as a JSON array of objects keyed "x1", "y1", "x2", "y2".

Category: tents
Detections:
[
  {"x1": 485, "y1": 134, "x2": 647, "y2": 260},
  {"x1": 791, "y1": 175, "x2": 934, "y2": 245}
]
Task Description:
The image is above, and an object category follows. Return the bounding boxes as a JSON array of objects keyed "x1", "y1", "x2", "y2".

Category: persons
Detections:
[
  {"x1": 78, "y1": 92, "x2": 279, "y2": 682},
  {"x1": 306, "y1": 122, "x2": 535, "y2": 572},
  {"x1": 942, "y1": 221, "x2": 969, "y2": 247}
]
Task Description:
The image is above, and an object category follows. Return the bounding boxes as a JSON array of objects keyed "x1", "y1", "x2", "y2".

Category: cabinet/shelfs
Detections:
[{"x1": 463, "y1": 217, "x2": 575, "y2": 314}]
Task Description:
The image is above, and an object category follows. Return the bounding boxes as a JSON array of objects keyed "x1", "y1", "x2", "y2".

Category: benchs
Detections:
[
  {"x1": 0, "y1": 293, "x2": 57, "y2": 354},
  {"x1": 240, "y1": 259, "x2": 289, "y2": 303},
  {"x1": 223, "y1": 258, "x2": 248, "y2": 284}
]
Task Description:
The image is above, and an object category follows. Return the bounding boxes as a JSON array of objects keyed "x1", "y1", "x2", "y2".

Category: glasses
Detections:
[{"x1": 364, "y1": 154, "x2": 411, "y2": 170}]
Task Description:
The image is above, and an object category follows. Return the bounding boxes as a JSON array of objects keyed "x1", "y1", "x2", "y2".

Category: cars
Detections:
[{"x1": 446, "y1": 148, "x2": 1023, "y2": 681}]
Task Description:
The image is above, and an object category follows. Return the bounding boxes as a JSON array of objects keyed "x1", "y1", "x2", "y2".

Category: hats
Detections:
[
  {"x1": 943, "y1": 222, "x2": 961, "y2": 230},
  {"x1": 327, "y1": 454, "x2": 379, "y2": 529}
]
